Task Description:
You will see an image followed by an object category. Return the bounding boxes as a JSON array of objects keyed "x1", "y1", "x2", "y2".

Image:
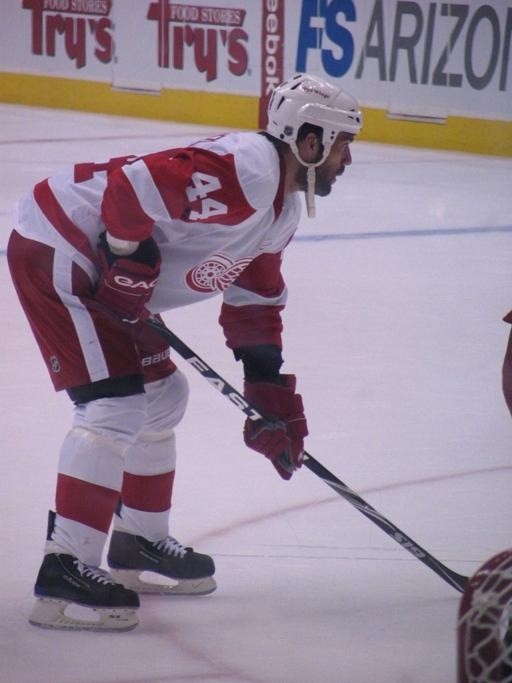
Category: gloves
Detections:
[
  {"x1": 92, "y1": 233, "x2": 161, "y2": 328},
  {"x1": 243, "y1": 373, "x2": 309, "y2": 481}
]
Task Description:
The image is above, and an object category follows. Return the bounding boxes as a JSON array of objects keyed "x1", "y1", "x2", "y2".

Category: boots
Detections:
[
  {"x1": 34, "y1": 553, "x2": 140, "y2": 609},
  {"x1": 107, "y1": 530, "x2": 216, "y2": 579}
]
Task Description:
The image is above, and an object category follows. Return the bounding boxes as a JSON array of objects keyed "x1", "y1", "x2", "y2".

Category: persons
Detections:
[{"x1": 7, "y1": 72, "x2": 362, "y2": 611}]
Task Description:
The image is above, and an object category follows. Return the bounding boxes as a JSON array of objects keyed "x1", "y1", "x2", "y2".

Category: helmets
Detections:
[{"x1": 265, "y1": 72, "x2": 363, "y2": 146}]
